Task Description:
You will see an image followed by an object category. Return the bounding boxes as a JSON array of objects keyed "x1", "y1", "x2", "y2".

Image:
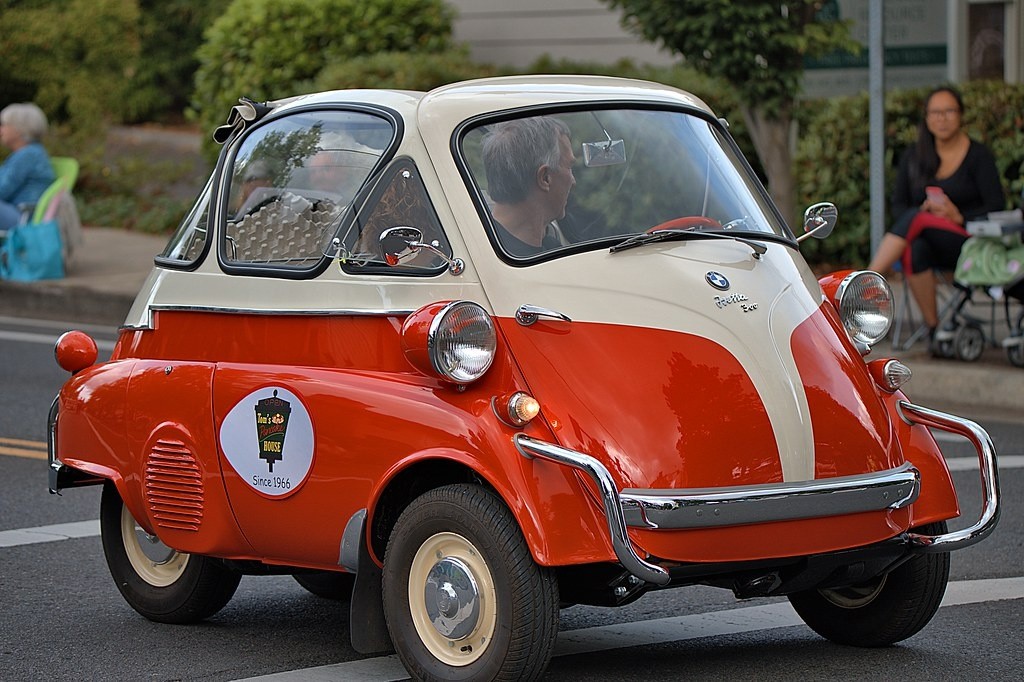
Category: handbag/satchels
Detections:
[{"x1": 0, "y1": 219, "x2": 66, "y2": 282}]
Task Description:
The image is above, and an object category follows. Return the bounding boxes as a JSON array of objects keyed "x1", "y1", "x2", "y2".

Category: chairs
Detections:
[
  {"x1": 0, "y1": 155, "x2": 78, "y2": 237},
  {"x1": 893, "y1": 262, "x2": 1003, "y2": 351}
]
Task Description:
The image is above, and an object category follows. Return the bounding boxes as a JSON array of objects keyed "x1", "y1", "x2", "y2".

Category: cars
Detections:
[{"x1": 47, "y1": 74, "x2": 1002, "y2": 682}]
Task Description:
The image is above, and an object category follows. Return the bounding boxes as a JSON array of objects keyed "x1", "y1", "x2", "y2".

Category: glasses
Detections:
[{"x1": 929, "y1": 108, "x2": 957, "y2": 120}]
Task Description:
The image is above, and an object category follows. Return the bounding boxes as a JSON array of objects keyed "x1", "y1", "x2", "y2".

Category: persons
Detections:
[
  {"x1": 863, "y1": 84, "x2": 1009, "y2": 356},
  {"x1": 240, "y1": 159, "x2": 279, "y2": 217},
  {"x1": 0, "y1": 102, "x2": 58, "y2": 235},
  {"x1": 480, "y1": 115, "x2": 578, "y2": 258},
  {"x1": 306, "y1": 147, "x2": 352, "y2": 195}
]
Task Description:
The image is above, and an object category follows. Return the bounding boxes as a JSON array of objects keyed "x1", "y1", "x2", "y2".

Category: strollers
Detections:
[{"x1": 929, "y1": 211, "x2": 1023, "y2": 367}]
